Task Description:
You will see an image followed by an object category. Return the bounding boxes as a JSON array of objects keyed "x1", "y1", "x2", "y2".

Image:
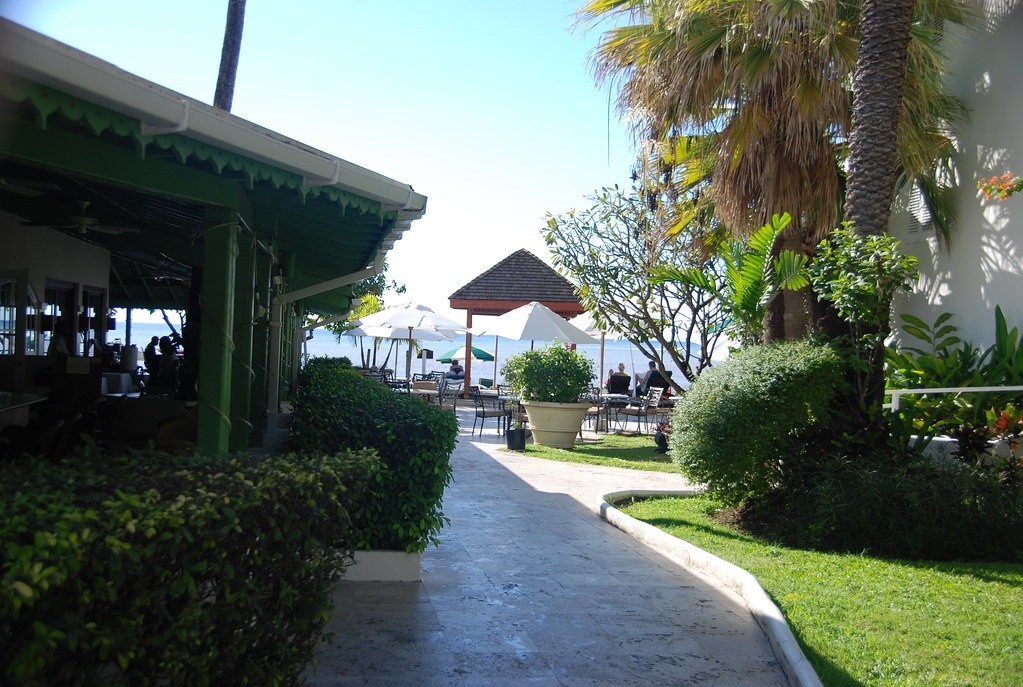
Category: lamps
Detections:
[{"x1": 654, "y1": 431, "x2": 670, "y2": 454}]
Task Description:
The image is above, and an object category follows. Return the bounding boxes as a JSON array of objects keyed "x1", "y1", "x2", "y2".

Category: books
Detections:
[{"x1": 637, "y1": 372, "x2": 645, "y2": 378}]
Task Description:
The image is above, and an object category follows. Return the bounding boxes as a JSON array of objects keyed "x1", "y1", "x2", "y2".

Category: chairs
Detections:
[
  {"x1": 470, "y1": 386, "x2": 518, "y2": 437},
  {"x1": 584, "y1": 371, "x2": 684, "y2": 435},
  {"x1": 358, "y1": 368, "x2": 465, "y2": 415},
  {"x1": 23, "y1": 393, "x2": 128, "y2": 457}
]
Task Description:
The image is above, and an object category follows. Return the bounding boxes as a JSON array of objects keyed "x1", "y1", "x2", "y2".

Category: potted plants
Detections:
[{"x1": 499, "y1": 337, "x2": 596, "y2": 450}]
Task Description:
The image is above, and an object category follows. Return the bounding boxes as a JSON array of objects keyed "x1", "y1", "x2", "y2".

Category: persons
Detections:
[
  {"x1": 47, "y1": 320, "x2": 71, "y2": 356},
  {"x1": 635, "y1": 361, "x2": 657, "y2": 387},
  {"x1": 160, "y1": 336, "x2": 177, "y2": 360},
  {"x1": 445, "y1": 360, "x2": 465, "y2": 379},
  {"x1": 604, "y1": 363, "x2": 627, "y2": 389},
  {"x1": 144, "y1": 336, "x2": 160, "y2": 366}
]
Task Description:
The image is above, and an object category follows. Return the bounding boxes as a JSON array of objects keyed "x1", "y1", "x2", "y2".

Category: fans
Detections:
[
  {"x1": 0, "y1": 177, "x2": 60, "y2": 199},
  {"x1": 21, "y1": 199, "x2": 142, "y2": 235}
]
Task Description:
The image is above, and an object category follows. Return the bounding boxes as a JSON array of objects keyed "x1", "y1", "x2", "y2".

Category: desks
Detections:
[
  {"x1": 478, "y1": 390, "x2": 512, "y2": 397},
  {"x1": 395, "y1": 389, "x2": 439, "y2": 403},
  {"x1": 586, "y1": 393, "x2": 628, "y2": 432},
  {"x1": 497, "y1": 396, "x2": 525, "y2": 429},
  {"x1": 105, "y1": 390, "x2": 200, "y2": 432},
  {"x1": 100, "y1": 371, "x2": 132, "y2": 393}
]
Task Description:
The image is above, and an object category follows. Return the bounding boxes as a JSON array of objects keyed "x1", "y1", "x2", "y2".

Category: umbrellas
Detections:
[
  {"x1": 467, "y1": 302, "x2": 602, "y2": 352},
  {"x1": 436, "y1": 344, "x2": 494, "y2": 364},
  {"x1": 568, "y1": 292, "x2": 744, "y2": 396},
  {"x1": 346, "y1": 300, "x2": 469, "y2": 395},
  {"x1": 342, "y1": 327, "x2": 461, "y2": 381}
]
